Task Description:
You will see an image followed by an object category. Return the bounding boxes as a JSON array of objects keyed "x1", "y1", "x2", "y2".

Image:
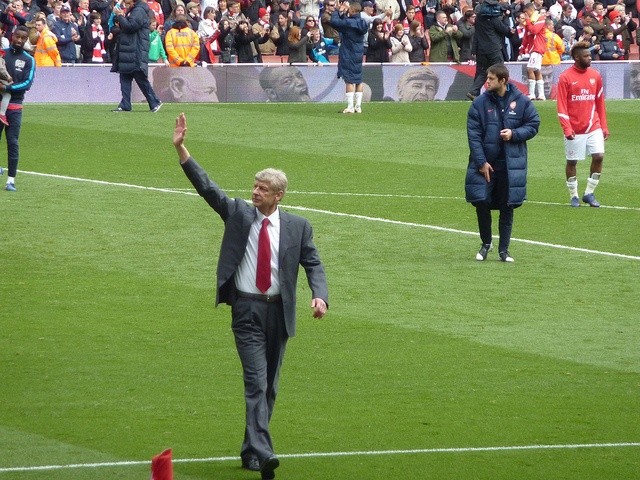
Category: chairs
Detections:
[
  {"x1": 261, "y1": 53, "x2": 366, "y2": 65},
  {"x1": 627, "y1": 17, "x2": 640, "y2": 60}
]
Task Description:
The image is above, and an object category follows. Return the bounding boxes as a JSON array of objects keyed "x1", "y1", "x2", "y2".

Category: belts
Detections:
[{"x1": 241, "y1": 293, "x2": 280, "y2": 303}]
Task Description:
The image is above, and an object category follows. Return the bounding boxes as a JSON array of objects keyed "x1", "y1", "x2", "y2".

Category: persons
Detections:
[
  {"x1": 172, "y1": 113, "x2": 330, "y2": 478},
  {"x1": 539, "y1": 8, "x2": 546, "y2": 19},
  {"x1": 455, "y1": 0, "x2": 517, "y2": 100},
  {"x1": 398, "y1": 68, "x2": 440, "y2": 101},
  {"x1": 260, "y1": 65, "x2": 311, "y2": 101},
  {"x1": 464, "y1": 62, "x2": 539, "y2": 263},
  {"x1": 557, "y1": 41, "x2": 610, "y2": 207},
  {"x1": 543, "y1": 0, "x2": 639, "y2": 63},
  {"x1": 165, "y1": 1, "x2": 221, "y2": 65},
  {"x1": 109, "y1": 0, "x2": 164, "y2": 113},
  {"x1": 1, "y1": 26, "x2": 36, "y2": 191},
  {"x1": 0, "y1": 49, "x2": 15, "y2": 127},
  {"x1": 221, "y1": 0, "x2": 338, "y2": 63},
  {"x1": 521, "y1": 2, "x2": 547, "y2": 100},
  {"x1": 148, "y1": 19, "x2": 170, "y2": 65},
  {"x1": 363, "y1": 0, "x2": 461, "y2": 63},
  {"x1": 459, "y1": 9, "x2": 478, "y2": 61},
  {"x1": 630, "y1": 62, "x2": 640, "y2": 99},
  {"x1": 532, "y1": 0, "x2": 543, "y2": 10},
  {"x1": 515, "y1": 12, "x2": 526, "y2": 47},
  {"x1": 153, "y1": 66, "x2": 220, "y2": 103},
  {"x1": 6, "y1": 0, "x2": 33, "y2": 26},
  {"x1": 331, "y1": 1, "x2": 369, "y2": 114},
  {"x1": 148, "y1": 0, "x2": 164, "y2": 28},
  {"x1": 522, "y1": 63, "x2": 553, "y2": 98},
  {"x1": 34, "y1": 0, "x2": 109, "y2": 66},
  {"x1": 24, "y1": 0, "x2": 33, "y2": 13}
]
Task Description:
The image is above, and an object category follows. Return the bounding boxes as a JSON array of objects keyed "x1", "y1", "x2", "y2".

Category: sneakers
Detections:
[
  {"x1": 151, "y1": 101, "x2": 164, "y2": 113},
  {"x1": 466, "y1": 92, "x2": 479, "y2": 101},
  {"x1": 0, "y1": 114, "x2": 9, "y2": 126},
  {"x1": 354, "y1": 106, "x2": 362, "y2": 113},
  {"x1": 583, "y1": 192, "x2": 601, "y2": 207},
  {"x1": 343, "y1": 108, "x2": 355, "y2": 113},
  {"x1": 476, "y1": 242, "x2": 493, "y2": 261},
  {"x1": 528, "y1": 94, "x2": 537, "y2": 100},
  {"x1": 571, "y1": 194, "x2": 580, "y2": 207},
  {"x1": 111, "y1": 107, "x2": 126, "y2": 112},
  {"x1": 500, "y1": 250, "x2": 515, "y2": 262},
  {"x1": 6, "y1": 181, "x2": 17, "y2": 191},
  {"x1": 538, "y1": 95, "x2": 546, "y2": 100}
]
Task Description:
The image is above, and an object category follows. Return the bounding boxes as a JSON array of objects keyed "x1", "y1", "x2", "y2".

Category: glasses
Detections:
[
  {"x1": 330, "y1": 4, "x2": 336, "y2": 7},
  {"x1": 617, "y1": 15, "x2": 622, "y2": 19},
  {"x1": 95, "y1": 18, "x2": 102, "y2": 21},
  {"x1": 308, "y1": 19, "x2": 315, "y2": 22}
]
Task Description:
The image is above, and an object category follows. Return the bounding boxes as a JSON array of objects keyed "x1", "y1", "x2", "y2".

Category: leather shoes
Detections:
[
  {"x1": 262, "y1": 456, "x2": 280, "y2": 480},
  {"x1": 242, "y1": 458, "x2": 260, "y2": 471}
]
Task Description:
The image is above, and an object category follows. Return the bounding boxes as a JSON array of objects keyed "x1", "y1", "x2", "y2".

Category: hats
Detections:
[
  {"x1": 362, "y1": 1, "x2": 376, "y2": 7},
  {"x1": 610, "y1": 11, "x2": 622, "y2": 21},
  {"x1": 187, "y1": 2, "x2": 201, "y2": 10},
  {"x1": 280, "y1": 0, "x2": 291, "y2": 5},
  {"x1": 563, "y1": 26, "x2": 577, "y2": 40},
  {"x1": 60, "y1": 7, "x2": 70, "y2": 11},
  {"x1": 258, "y1": 8, "x2": 270, "y2": 18}
]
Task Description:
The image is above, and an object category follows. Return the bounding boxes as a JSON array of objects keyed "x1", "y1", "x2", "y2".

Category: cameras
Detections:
[{"x1": 481, "y1": 0, "x2": 517, "y2": 16}]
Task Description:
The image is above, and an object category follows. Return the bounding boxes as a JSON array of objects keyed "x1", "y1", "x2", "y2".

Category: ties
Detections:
[{"x1": 256, "y1": 218, "x2": 272, "y2": 294}]
